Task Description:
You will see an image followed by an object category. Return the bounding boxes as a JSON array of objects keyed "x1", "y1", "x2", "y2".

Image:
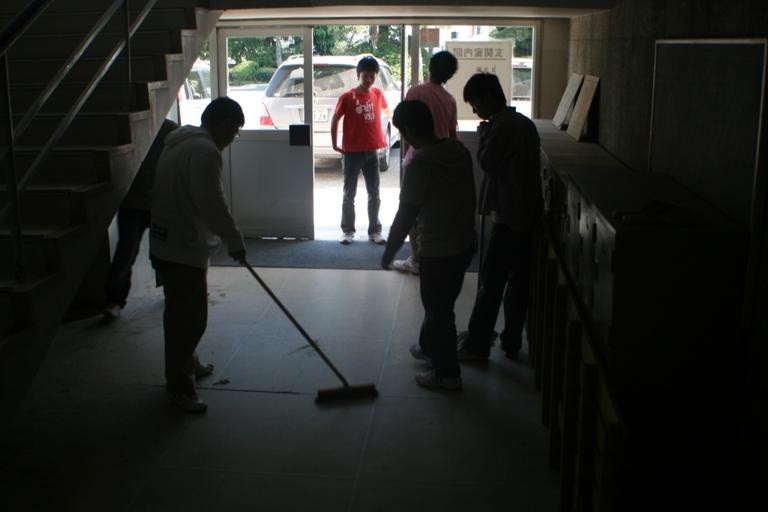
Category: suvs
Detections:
[{"x1": 258, "y1": 53, "x2": 402, "y2": 170}]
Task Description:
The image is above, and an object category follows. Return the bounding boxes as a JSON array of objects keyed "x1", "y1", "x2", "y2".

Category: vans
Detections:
[{"x1": 168, "y1": 58, "x2": 211, "y2": 128}]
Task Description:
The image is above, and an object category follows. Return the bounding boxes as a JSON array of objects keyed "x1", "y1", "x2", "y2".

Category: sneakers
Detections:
[
  {"x1": 339, "y1": 233, "x2": 353, "y2": 243},
  {"x1": 173, "y1": 399, "x2": 206, "y2": 414},
  {"x1": 409, "y1": 330, "x2": 518, "y2": 390},
  {"x1": 368, "y1": 232, "x2": 386, "y2": 243},
  {"x1": 192, "y1": 363, "x2": 214, "y2": 378},
  {"x1": 392, "y1": 256, "x2": 419, "y2": 276}
]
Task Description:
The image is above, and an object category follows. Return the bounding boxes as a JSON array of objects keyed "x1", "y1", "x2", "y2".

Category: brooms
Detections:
[{"x1": 238, "y1": 257, "x2": 380, "y2": 405}]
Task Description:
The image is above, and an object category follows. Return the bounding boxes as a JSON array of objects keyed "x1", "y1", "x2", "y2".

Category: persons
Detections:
[
  {"x1": 458, "y1": 71, "x2": 544, "y2": 362},
  {"x1": 379, "y1": 97, "x2": 477, "y2": 391},
  {"x1": 394, "y1": 52, "x2": 457, "y2": 275},
  {"x1": 150, "y1": 97, "x2": 250, "y2": 417},
  {"x1": 328, "y1": 57, "x2": 394, "y2": 245},
  {"x1": 101, "y1": 119, "x2": 179, "y2": 321}
]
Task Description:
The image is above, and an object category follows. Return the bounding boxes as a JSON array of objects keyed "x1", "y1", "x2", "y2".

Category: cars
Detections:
[{"x1": 511, "y1": 57, "x2": 532, "y2": 119}]
